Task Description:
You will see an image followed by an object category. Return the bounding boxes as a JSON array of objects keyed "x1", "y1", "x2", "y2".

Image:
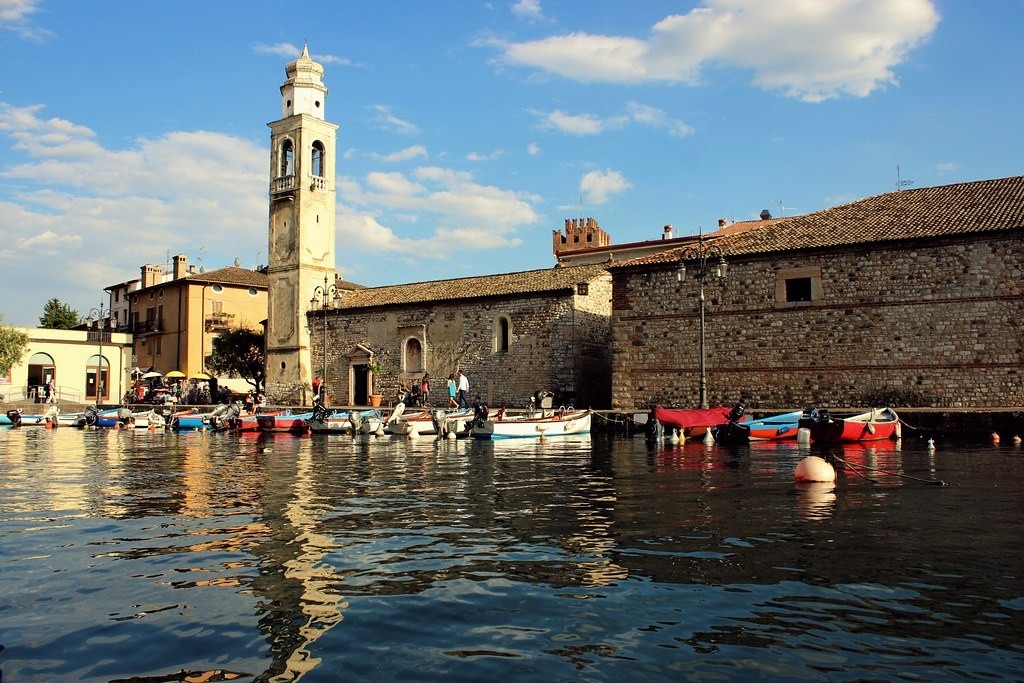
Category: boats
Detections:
[
  {"x1": 716, "y1": 408, "x2": 803, "y2": 442},
  {"x1": 0, "y1": 402, "x2": 489, "y2": 434},
  {"x1": 643, "y1": 404, "x2": 745, "y2": 441},
  {"x1": 800, "y1": 406, "x2": 900, "y2": 443},
  {"x1": 468, "y1": 400, "x2": 592, "y2": 440}
]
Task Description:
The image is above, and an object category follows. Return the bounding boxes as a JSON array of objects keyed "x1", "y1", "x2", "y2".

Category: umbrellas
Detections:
[
  {"x1": 165, "y1": 371, "x2": 185, "y2": 380},
  {"x1": 190, "y1": 371, "x2": 210, "y2": 383},
  {"x1": 142, "y1": 371, "x2": 164, "y2": 390}
]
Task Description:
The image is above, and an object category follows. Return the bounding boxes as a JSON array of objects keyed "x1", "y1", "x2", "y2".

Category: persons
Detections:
[
  {"x1": 46, "y1": 379, "x2": 58, "y2": 404},
  {"x1": 135, "y1": 379, "x2": 232, "y2": 404},
  {"x1": 312, "y1": 376, "x2": 323, "y2": 406},
  {"x1": 101, "y1": 378, "x2": 105, "y2": 396},
  {"x1": 447, "y1": 370, "x2": 472, "y2": 410},
  {"x1": 398, "y1": 373, "x2": 430, "y2": 407},
  {"x1": 246, "y1": 391, "x2": 266, "y2": 415}
]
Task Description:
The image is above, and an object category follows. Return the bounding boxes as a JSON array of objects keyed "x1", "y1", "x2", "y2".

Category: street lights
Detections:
[
  {"x1": 311, "y1": 275, "x2": 341, "y2": 408},
  {"x1": 676, "y1": 226, "x2": 728, "y2": 409},
  {"x1": 87, "y1": 296, "x2": 117, "y2": 403}
]
